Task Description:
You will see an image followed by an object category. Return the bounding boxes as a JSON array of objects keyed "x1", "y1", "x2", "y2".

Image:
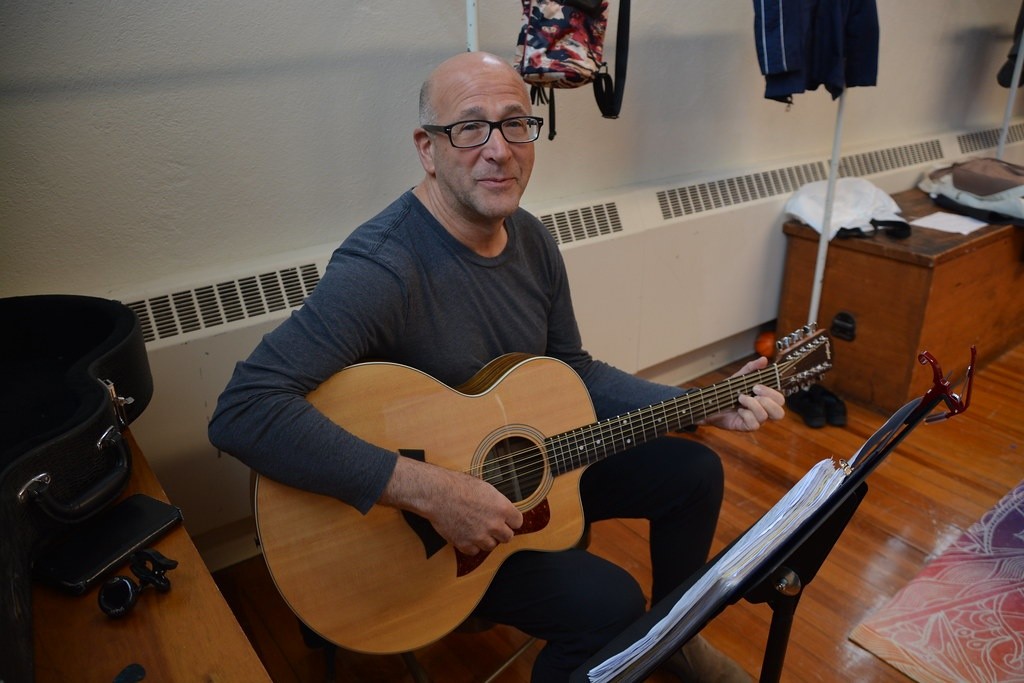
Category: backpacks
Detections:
[{"x1": 514, "y1": 0, "x2": 631, "y2": 141}]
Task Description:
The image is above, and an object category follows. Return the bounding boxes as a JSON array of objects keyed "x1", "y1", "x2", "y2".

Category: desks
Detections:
[{"x1": 34, "y1": 427, "x2": 275, "y2": 683}]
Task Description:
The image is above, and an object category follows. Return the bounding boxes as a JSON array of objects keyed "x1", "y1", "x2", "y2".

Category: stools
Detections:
[{"x1": 321, "y1": 522, "x2": 590, "y2": 683}]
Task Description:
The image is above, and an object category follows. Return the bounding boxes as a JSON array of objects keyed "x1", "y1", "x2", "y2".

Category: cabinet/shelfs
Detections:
[{"x1": 775, "y1": 188, "x2": 1024, "y2": 418}]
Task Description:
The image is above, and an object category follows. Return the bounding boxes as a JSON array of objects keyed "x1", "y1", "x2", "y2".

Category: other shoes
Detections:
[
  {"x1": 658, "y1": 634, "x2": 754, "y2": 683},
  {"x1": 785, "y1": 383, "x2": 848, "y2": 426}
]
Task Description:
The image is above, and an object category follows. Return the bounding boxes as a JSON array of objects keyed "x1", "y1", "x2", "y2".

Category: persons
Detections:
[{"x1": 206, "y1": 52, "x2": 787, "y2": 683}]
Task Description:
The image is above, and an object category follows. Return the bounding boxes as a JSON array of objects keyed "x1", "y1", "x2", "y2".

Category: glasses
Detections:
[{"x1": 421, "y1": 116, "x2": 544, "y2": 148}]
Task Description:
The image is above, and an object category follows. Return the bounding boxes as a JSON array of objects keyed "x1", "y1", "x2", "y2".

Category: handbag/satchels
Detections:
[{"x1": 953, "y1": 157, "x2": 1024, "y2": 196}]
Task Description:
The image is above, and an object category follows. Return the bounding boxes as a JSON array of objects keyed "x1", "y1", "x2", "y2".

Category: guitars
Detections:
[{"x1": 251, "y1": 320, "x2": 838, "y2": 657}]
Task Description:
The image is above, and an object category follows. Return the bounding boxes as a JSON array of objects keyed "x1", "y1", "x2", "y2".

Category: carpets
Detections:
[{"x1": 849, "y1": 482, "x2": 1024, "y2": 683}]
